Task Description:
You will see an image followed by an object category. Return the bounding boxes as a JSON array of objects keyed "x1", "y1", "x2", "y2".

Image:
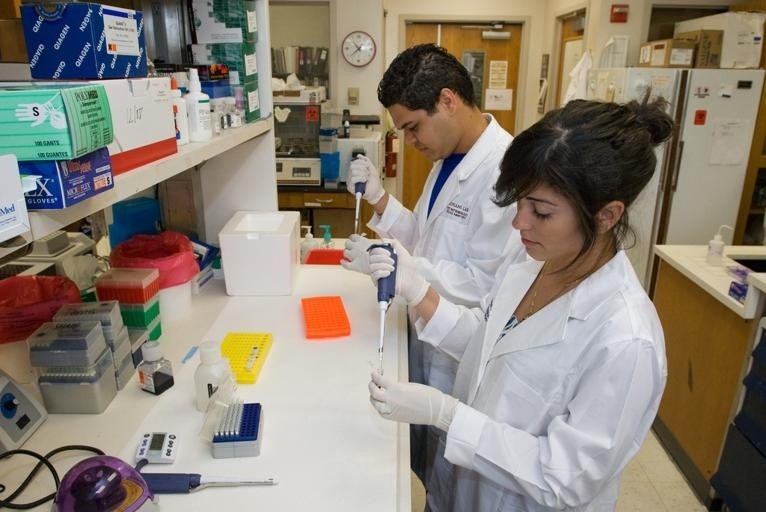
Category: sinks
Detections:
[{"x1": 735, "y1": 259, "x2": 766, "y2": 272}]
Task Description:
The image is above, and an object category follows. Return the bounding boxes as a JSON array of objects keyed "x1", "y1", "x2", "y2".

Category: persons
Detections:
[
  {"x1": 367, "y1": 86, "x2": 677, "y2": 512},
  {"x1": 338, "y1": 42, "x2": 528, "y2": 493}
]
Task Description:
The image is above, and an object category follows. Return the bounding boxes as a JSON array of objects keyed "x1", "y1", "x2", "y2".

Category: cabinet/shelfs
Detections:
[
  {"x1": 278, "y1": 178, "x2": 375, "y2": 239},
  {"x1": 728, "y1": 1, "x2": 765, "y2": 245}
]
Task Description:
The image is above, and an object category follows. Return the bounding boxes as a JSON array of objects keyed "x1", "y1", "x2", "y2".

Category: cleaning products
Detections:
[{"x1": 707, "y1": 225, "x2": 733, "y2": 263}]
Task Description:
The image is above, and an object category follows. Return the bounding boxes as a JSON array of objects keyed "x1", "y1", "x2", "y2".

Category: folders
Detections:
[{"x1": 299, "y1": 47, "x2": 328, "y2": 79}]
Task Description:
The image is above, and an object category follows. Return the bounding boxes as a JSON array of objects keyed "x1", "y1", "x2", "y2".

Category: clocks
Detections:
[{"x1": 342, "y1": 32, "x2": 376, "y2": 67}]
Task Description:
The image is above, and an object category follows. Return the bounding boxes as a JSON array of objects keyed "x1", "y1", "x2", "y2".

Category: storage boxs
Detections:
[
  {"x1": 189, "y1": 0, "x2": 260, "y2": 123},
  {"x1": 18, "y1": 1, "x2": 146, "y2": 81},
  {"x1": 636, "y1": 9, "x2": 765, "y2": 68},
  {"x1": 49, "y1": 79, "x2": 176, "y2": 179},
  {"x1": 0, "y1": 85, "x2": 114, "y2": 245},
  {"x1": 106, "y1": 197, "x2": 158, "y2": 255}
]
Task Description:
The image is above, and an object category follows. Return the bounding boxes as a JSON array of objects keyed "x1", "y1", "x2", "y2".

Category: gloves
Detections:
[
  {"x1": 345, "y1": 153, "x2": 385, "y2": 206},
  {"x1": 340, "y1": 235, "x2": 431, "y2": 307},
  {"x1": 369, "y1": 370, "x2": 460, "y2": 434}
]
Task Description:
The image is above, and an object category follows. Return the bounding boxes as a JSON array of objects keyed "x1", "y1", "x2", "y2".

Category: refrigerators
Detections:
[{"x1": 615, "y1": 65, "x2": 766, "y2": 293}]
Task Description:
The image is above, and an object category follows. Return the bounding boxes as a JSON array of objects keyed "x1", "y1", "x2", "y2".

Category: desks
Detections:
[{"x1": 0, "y1": 0, "x2": 415, "y2": 512}]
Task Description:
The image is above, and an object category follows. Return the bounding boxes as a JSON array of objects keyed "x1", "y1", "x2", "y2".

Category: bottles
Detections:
[
  {"x1": 184, "y1": 68, "x2": 212, "y2": 144},
  {"x1": 192, "y1": 337, "x2": 234, "y2": 413},
  {"x1": 171, "y1": 77, "x2": 189, "y2": 147},
  {"x1": 706, "y1": 234, "x2": 726, "y2": 266},
  {"x1": 136, "y1": 340, "x2": 174, "y2": 395}
]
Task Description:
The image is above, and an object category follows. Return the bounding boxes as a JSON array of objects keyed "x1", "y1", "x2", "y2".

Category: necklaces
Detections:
[{"x1": 523, "y1": 256, "x2": 588, "y2": 319}]
